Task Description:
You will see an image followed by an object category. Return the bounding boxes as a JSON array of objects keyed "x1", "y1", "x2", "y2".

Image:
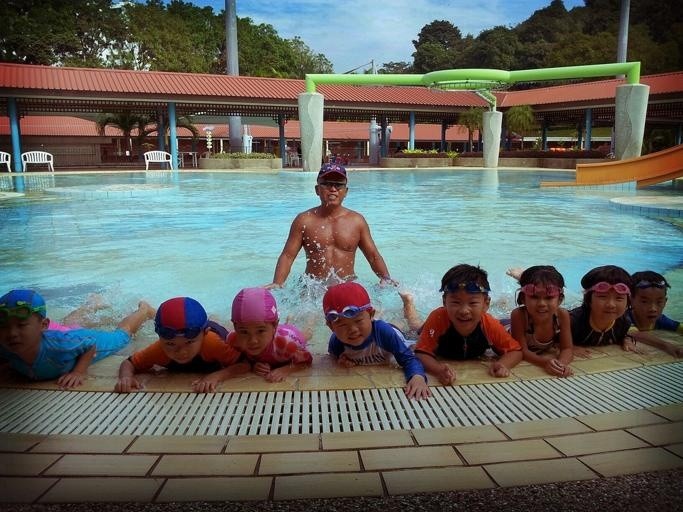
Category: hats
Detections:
[
  {"x1": 0, "y1": 290, "x2": 46, "y2": 318},
  {"x1": 317, "y1": 163, "x2": 347, "y2": 184},
  {"x1": 323, "y1": 281, "x2": 372, "y2": 322},
  {"x1": 155, "y1": 297, "x2": 208, "y2": 336},
  {"x1": 232, "y1": 288, "x2": 278, "y2": 323}
]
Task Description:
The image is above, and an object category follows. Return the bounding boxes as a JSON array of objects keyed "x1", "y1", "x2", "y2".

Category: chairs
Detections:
[
  {"x1": 0, "y1": 152, "x2": 12, "y2": 173},
  {"x1": 21, "y1": 151, "x2": 56, "y2": 172},
  {"x1": 144, "y1": 151, "x2": 173, "y2": 170},
  {"x1": 288, "y1": 151, "x2": 300, "y2": 168}
]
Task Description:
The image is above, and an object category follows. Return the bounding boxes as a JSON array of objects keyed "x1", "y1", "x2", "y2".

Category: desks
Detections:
[{"x1": 181, "y1": 151, "x2": 198, "y2": 168}]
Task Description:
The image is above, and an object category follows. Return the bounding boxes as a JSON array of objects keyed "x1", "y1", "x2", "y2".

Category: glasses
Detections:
[
  {"x1": 439, "y1": 281, "x2": 488, "y2": 294},
  {"x1": 0, "y1": 303, "x2": 46, "y2": 322},
  {"x1": 521, "y1": 284, "x2": 563, "y2": 296},
  {"x1": 324, "y1": 302, "x2": 371, "y2": 322},
  {"x1": 155, "y1": 321, "x2": 209, "y2": 340},
  {"x1": 320, "y1": 180, "x2": 347, "y2": 190},
  {"x1": 584, "y1": 282, "x2": 632, "y2": 301},
  {"x1": 634, "y1": 279, "x2": 666, "y2": 290}
]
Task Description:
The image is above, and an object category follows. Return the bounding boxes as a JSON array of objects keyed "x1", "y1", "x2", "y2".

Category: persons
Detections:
[
  {"x1": 113, "y1": 295, "x2": 251, "y2": 395},
  {"x1": 226, "y1": 286, "x2": 319, "y2": 384},
  {"x1": 497, "y1": 265, "x2": 575, "y2": 378},
  {"x1": 397, "y1": 261, "x2": 525, "y2": 386},
  {"x1": 570, "y1": 264, "x2": 647, "y2": 359},
  {"x1": 263, "y1": 163, "x2": 399, "y2": 298},
  {"x1": 505, "y1": 267, "x2": 683, "y2": 358},
  {"x1": 0, "y1": 288, "x2": 157, "y2": 389},
  {"x1": 321, "y1": 281, "x2": 432, "y2": 402}
]
